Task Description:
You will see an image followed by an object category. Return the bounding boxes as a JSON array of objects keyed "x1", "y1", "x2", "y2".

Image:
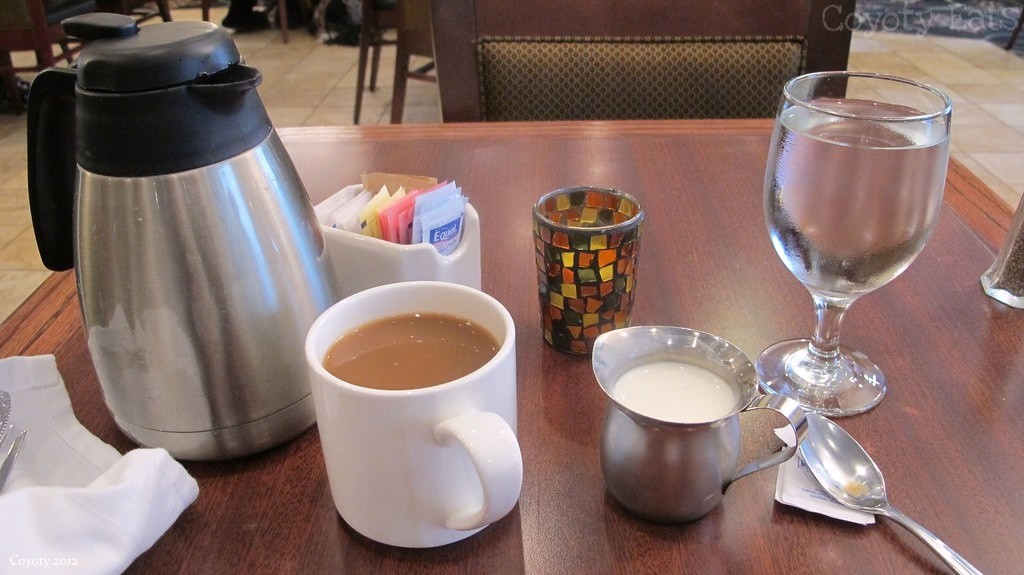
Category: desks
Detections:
[{"x1": 0, "y1": 120, "x2": 1024, "y2": 575}]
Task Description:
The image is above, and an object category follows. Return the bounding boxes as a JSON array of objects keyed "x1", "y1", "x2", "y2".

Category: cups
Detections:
[
  {"x1": 592, "y1": 326, "x2": 809, "y2": 522},
  {"x1": 533, "y1": 186, "x2": 643, "y2": 355},
  {"x1": 305, "y1": 281, "x2": 523, "y2": 548}
]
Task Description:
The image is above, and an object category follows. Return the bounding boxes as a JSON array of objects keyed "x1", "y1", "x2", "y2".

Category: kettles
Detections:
[{"x1": 27, "y1": 13, "x2": 339, "y2": 460}]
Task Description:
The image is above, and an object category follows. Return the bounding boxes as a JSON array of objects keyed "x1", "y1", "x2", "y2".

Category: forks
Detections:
[{"x1": 0, "y1": 423, "x2": 26, "y2": 491}]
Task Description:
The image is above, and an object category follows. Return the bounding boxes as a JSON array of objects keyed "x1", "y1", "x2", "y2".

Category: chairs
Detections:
[{"x1": 429, "y1": 0, "x2": 855, "y2": 125}]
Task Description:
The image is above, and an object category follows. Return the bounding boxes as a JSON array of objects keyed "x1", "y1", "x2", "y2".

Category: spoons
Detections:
[{"x1": 800, "y1": 411, "x2": 984, "y2": 575}]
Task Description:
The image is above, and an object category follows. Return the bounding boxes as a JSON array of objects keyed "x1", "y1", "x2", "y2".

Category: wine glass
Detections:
[{"x1": 755, "y1": 71, "x2": 952, "y2": 418}]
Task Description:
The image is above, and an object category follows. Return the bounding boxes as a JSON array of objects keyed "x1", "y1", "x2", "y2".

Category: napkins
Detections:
[{"x1": 0, "y1": 351, "x2": 201, "y2": 575}]
